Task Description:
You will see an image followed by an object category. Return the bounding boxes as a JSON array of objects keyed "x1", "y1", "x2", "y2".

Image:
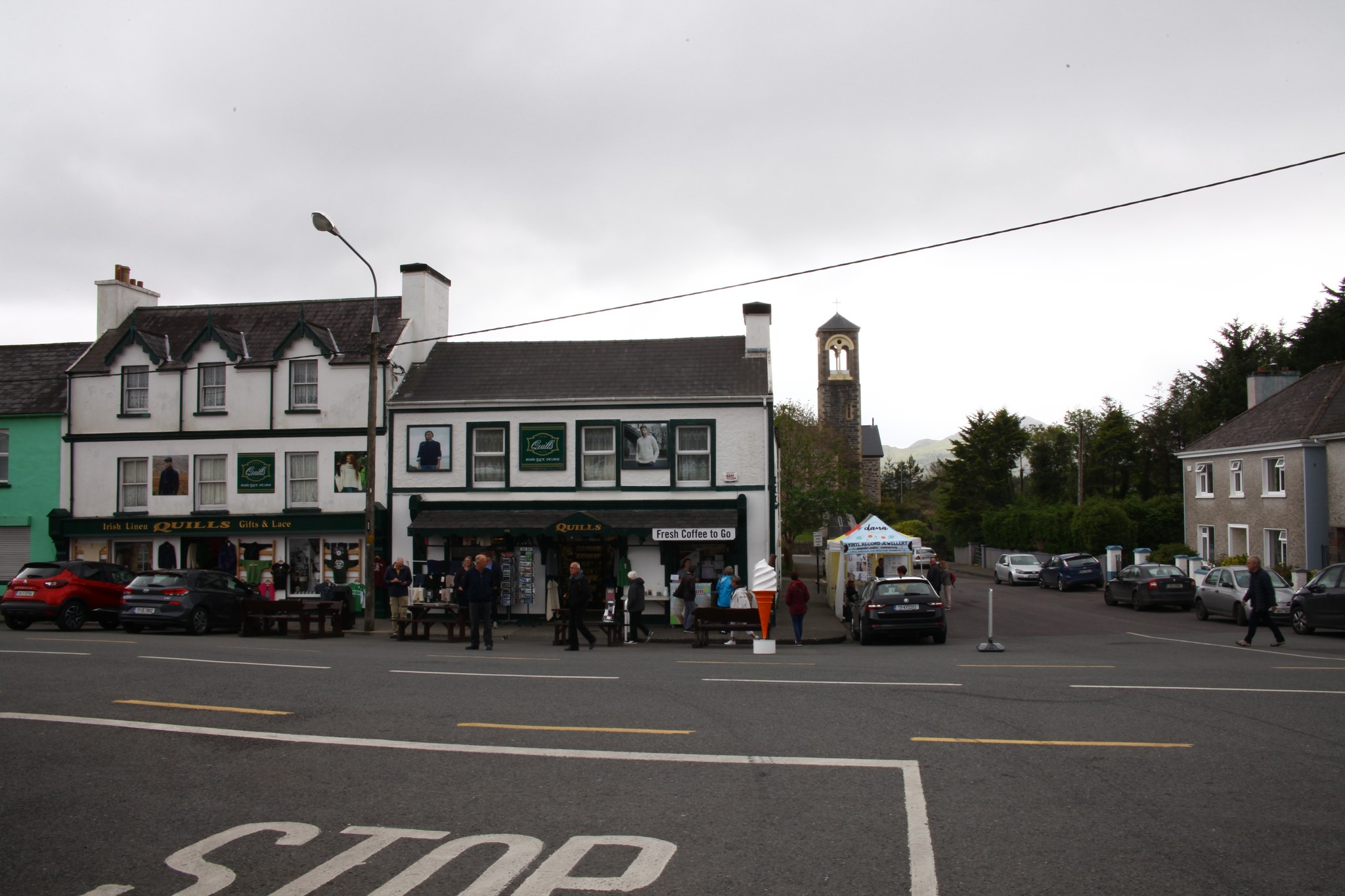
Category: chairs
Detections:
[{"x1": 907, "y1": 585, "x2": 917, "y2": 593}]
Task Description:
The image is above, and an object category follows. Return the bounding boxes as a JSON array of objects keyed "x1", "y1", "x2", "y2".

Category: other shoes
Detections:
[
  {"x1": 564, "y1": 646, "x2": 579, "y2": 651},
  {"x1": 465, "y1": 645, "x2": 479, "y2": 650},
  {"x1": 720, "y1": 631, "x2": 727, "y2": 635},
  {"x1": 945, "y1": 607, "x2": 951, "y2": 611},
  {"x1": 794, "y1": 640, "x2": 802, "y2": 646},
  {"x1": 493, "y1": 622, "x2": 498, "y2": 628},
  {"x1": 589, "y1": 637, "x2": 596, "y2": 650},
  {"x1": 684, "y1": 628, "x2": 695, "y2": 633},
  {"x1": 486, "y1": 644, "x2": 492, "y2": 650},
  {"x1": 624, "y1": 639, "x2": 638, "y2": 644},
  {"x1": 646, "y1": 631, "x2": 653, "y2": 642}
]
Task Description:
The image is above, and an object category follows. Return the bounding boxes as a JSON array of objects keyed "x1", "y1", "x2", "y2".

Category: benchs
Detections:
[
  {"x1": 548, "y1": 620, "x2": 619, "y2": 646},
  {"x1": 237, "y1": 598, "x2": 344, "y2": 637},
  {"x1": 689, "y1": 623, "x2": 770, "y2": 645},
  {"x1": 391, "y1": 618, "x2": 468, "y2": 641}
]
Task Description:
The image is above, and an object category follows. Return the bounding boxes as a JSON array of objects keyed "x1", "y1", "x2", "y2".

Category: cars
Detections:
[
  {"x1": 1104, "y1": 564, "x2": 1196, "y2": 612},
  {"x1": 1194, "y1": 564, "x2": 1297, "y2": 627},
  {"x1": 912, "y1": 548, "x2": 938, "y2": 569},
  {"x1": 1289, "y1": 563, "x2": 1345, "y2": 635},
  {"x1": 994, "y1": 554, "x2": 1044, "y2": 586}
]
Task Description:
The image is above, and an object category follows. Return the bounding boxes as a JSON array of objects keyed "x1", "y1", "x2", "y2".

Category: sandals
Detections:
[
  {"x1": 1270, "y1": 641, "x2": 1286, "y2": 647},
  {"x1": 1235, "y1": 641, "x2": 1251, "y2": 647}
]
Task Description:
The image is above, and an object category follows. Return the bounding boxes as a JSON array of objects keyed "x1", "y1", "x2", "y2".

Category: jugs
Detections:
[{"x1": 663, "y1": 587, "x2": 669, "y2": 597}]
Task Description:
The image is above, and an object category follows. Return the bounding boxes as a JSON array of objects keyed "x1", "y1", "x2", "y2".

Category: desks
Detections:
[
  {"x1": 621, "y1": 593, "x2": 670, "y2": 627},
  {"x1": 404, "y1": 605, "x2": 467, "y2": 641},
  {"x1": 552, "y1": 607, "x2": 627, "y2": 643},
  {"x1": 695, "y1": 607, "x2": 774, "y2": 648}
]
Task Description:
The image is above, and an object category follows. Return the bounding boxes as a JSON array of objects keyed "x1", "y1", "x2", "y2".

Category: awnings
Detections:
[{"x1": 407, "y1": 508, "x2": 741, "y2": 539}]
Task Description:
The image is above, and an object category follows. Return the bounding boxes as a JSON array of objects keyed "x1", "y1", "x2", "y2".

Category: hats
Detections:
[{"x1": 790, "y1": 572, "x2": 799, "y2": 580}]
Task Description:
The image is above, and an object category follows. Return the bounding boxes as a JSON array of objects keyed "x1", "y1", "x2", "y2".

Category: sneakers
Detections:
[
  {"x1": 724, "y1": 639, "x2": 736, "y2": 645},
  {"x1": 752, "y1": 636, "x2": 759, "y2": 645}
]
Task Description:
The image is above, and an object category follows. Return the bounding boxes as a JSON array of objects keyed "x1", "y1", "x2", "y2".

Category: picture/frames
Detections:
[{"x1": 406, "y1": 425, "x2": 452, "y2": 472}]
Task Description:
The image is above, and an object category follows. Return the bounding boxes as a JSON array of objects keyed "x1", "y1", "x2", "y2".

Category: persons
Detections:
[
  {"x1": 384, "y1": 558, "x2": 413, "y2": 638},
  {"x1": 716, "y1": 566, "x2": 735, "y2": 634},
  {"x1": 875, "y1": 558, "x2": 884, "y2": 578},
  {"x1": 453, "y1": 556, "x2": 474, "y2": 630},
  {"x1": 564, "y1": 562, "x2": 596, "y2": 651},
  {"x1": 334, "y1": 454, "x2": 365, "y2": 493},
  {"x1": 463, "y1": 554, "x2": 494, "y2": 650},
  {"x1": 681, "y1": 565, "x2": 697, "y2": 632},
  {"x1": 940, "y1": 561, "x2": 953, "y2": 610},
  {"x1": 1235, "y1": 555, "x2": 1285, "y2": 647},
  {"x1": 897, "y1": 565, "x2": 915, "y2": 592},
  {"x1": 624, "y1": 571, "x2": 654, "y2": 643},
  {"x1": 846, "y1": 576, "x2": 858, "y2": 603},
  {"x1": 676, "y1": 558, "x2": 700, "y2": 582},
  {"x1": 483, "y1": 551, "x2": 503, "y2": 628},
  {"x1": 417, "y1": 430, "x2": 442, "y2": 469},
  {"x1": 724, "y1": 575, "x2": 760, "y2": 645},
  {"x1": 926, "y1": 557, "x2": 941, "y2": 596},
  {"x1": 785, "y1": 571, "x2": 809, "y2": 646},
  {"x1": 158, "y1": 457, "x2": 179, "y2": 495},
  {"x1": 635, "y1": 425, "x2": 660, "y2": 467}
]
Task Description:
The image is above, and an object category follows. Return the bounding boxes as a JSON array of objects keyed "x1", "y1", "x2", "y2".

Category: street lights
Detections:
[{"x1": 311, "y1": 211, "x2": 380, "y2": 632}]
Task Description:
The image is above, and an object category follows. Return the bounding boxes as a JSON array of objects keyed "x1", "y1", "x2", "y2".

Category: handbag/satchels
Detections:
[
  {"x1": 745, "y1": 592, "x2": 753, "y2": 607},
  {"x1": 950, "y1": 572, "x2": 956, "y2": 584},
  {"x1": 673, "y1": 584, "x2": 683, "y2": 599}
]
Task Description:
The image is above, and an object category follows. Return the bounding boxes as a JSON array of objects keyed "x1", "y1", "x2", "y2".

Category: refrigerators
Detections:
[{"x1": 670, "y1": 583, "x2": 711, "y2": 628}]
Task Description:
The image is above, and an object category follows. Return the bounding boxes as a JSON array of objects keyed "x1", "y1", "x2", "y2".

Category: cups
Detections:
[
  {"x1": 607, "y1": 601, "x2": 614, "y2": 616},
  {"x1": 648, "y1": 589, "x2": 652, "y2": 596}
]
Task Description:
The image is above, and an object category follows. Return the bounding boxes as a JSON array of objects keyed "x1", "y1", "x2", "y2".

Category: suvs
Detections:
[
  {"x1": 0, "y1": 560, "x2": 139, "y2": 633},
  {"x1": 118, "y1": 567, "x2": 276, "y2": 636},
  {"x1": 850, "y1": 575, "x2": 947, "y2": 646},
  {"x1": 1038, "y1": 552, "x2": 1104, "y2": 592}
]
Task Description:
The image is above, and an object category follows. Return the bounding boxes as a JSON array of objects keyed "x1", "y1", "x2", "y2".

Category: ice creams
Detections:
[{"x1": 752, "y1": 554, "x2": 777, "y2": 639}]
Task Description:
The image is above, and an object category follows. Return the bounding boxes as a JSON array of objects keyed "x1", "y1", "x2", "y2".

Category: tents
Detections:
[
  {"x1": 835, "y1": 516, "x2": 913, "y2": 621},
  {"x1": 825, "y1": 514, "x2": 923, "y2": 610}
]
